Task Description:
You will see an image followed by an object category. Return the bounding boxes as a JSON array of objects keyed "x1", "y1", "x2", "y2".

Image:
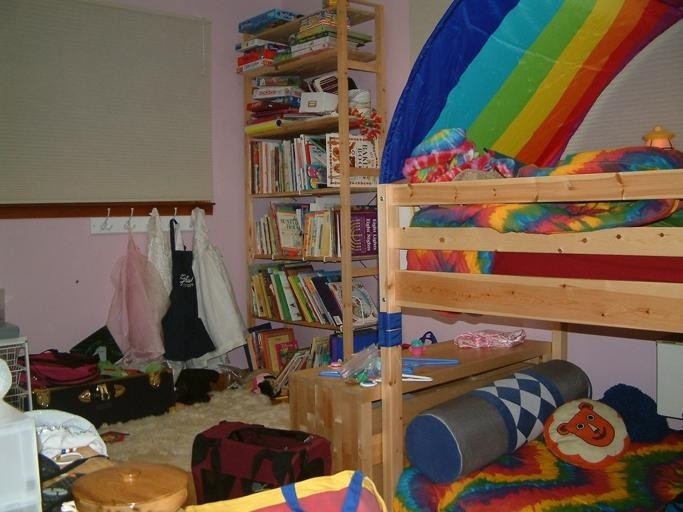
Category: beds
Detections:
[{"x1": 377, "y1": 168, "x2": 682, "y2": 512}]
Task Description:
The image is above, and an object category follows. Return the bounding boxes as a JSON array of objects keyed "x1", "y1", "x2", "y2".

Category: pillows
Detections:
[{"x1": 405, "y1": 359, "x2": 592, "y2": 484}]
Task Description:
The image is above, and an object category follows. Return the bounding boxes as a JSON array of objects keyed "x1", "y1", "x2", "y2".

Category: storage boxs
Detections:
[{"x1": 17, "y1": 365, "x2": 174, "y2": 428}]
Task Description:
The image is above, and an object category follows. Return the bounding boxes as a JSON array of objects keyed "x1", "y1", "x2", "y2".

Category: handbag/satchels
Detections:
[
  {"x1": 192, "y1": 415, "x2": 335, "y2": 506},
  {"x1": 14, "y1": 346, "x2": 102, "y2": 388}
]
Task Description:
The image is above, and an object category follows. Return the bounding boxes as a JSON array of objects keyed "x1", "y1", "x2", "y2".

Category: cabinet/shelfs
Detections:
[
  {"x1": 0, "y1": 335, "x2": 42, "y2": 512},
  {"x1": 240, "y1": 0, "x2": 387, "y2": 405},
  {"x1": 290, "y1": 328, "x2": 553, "y2": 502}
]
